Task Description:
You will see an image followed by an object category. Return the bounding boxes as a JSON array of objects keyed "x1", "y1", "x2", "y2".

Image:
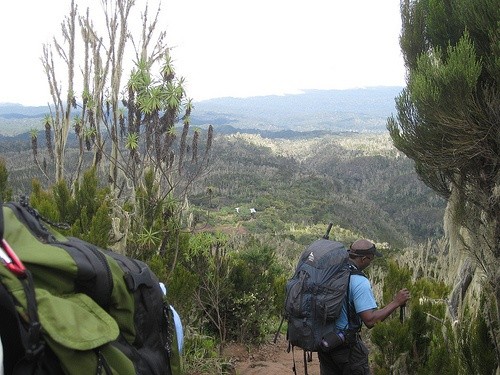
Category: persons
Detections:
[{"x1": 308, "y1": 240, "x2": 413, "y2": 375}]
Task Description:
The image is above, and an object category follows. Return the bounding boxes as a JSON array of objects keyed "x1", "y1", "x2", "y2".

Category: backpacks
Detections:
[
  {"x1": 272, "y1": 237, "x2": 364, "y2": 357},
  {"x1": 1, "y1": 200, "x2": 185, "y2": 375}
]
